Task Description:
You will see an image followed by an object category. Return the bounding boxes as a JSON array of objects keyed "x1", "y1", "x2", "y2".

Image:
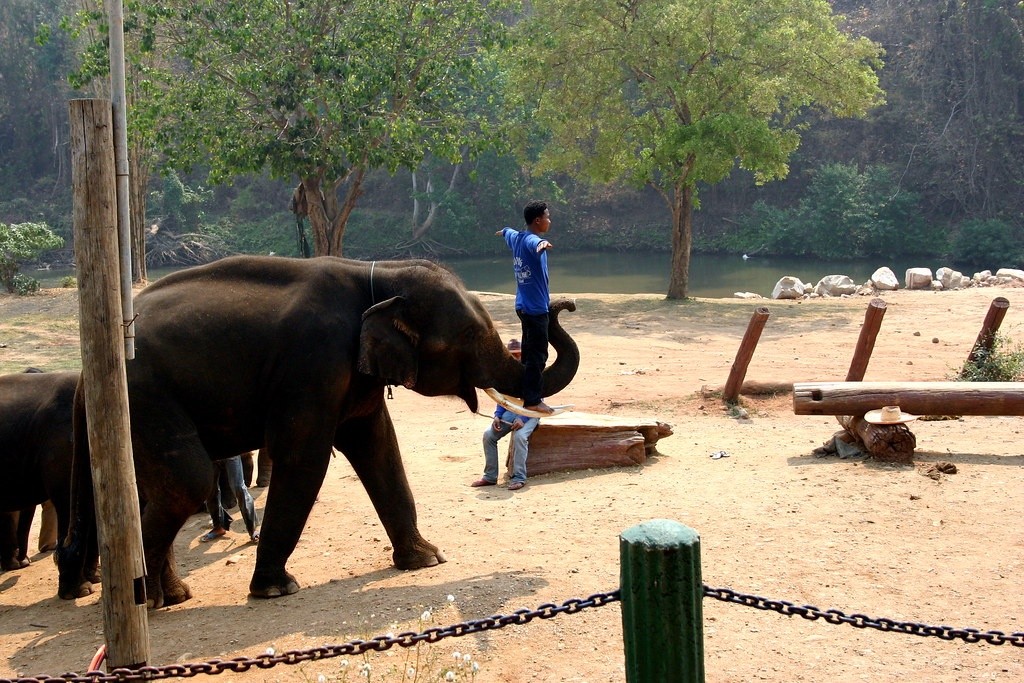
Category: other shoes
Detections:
[
  {"x1": 508, "y1": 481, "x2": 525, "y2": 490},
  {"x1": 472, "y1": 480, "x2": 496, "y2": 486}
]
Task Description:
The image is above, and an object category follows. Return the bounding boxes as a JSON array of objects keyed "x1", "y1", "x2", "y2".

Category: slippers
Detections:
[
  {"x1": 250, "y1": 532, "x2": 259, "y2": 543},
  {"x1": 202, "y1": 530, "x2": 226, "y2": 542}
]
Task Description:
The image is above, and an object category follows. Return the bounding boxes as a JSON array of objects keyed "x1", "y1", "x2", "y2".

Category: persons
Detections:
[
  {"x1": 201, "y1": 455, "x2": 261, "y2": 544},
  {"x1": 494, "y1": 199, "x2": 555, "y2": 414},
  {"x1": 24, "y1": 367, "x2": 57, "y2": 553},
  {"x1": 471, "y1": 339, "x2": 541, "y2": 490}
]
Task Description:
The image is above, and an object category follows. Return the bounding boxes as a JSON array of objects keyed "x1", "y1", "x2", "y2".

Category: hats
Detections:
[
  {"x1": 507, "y1": 339, "x2": 521, "y2": 353},
  {"x1": 865, "y1": 406, "x2": 915, "y2": 424}
]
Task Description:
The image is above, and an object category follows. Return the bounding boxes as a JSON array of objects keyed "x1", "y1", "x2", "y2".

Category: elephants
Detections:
[{"x1": 0, "y1": 254, "x2": 581, "y2": 611}]
